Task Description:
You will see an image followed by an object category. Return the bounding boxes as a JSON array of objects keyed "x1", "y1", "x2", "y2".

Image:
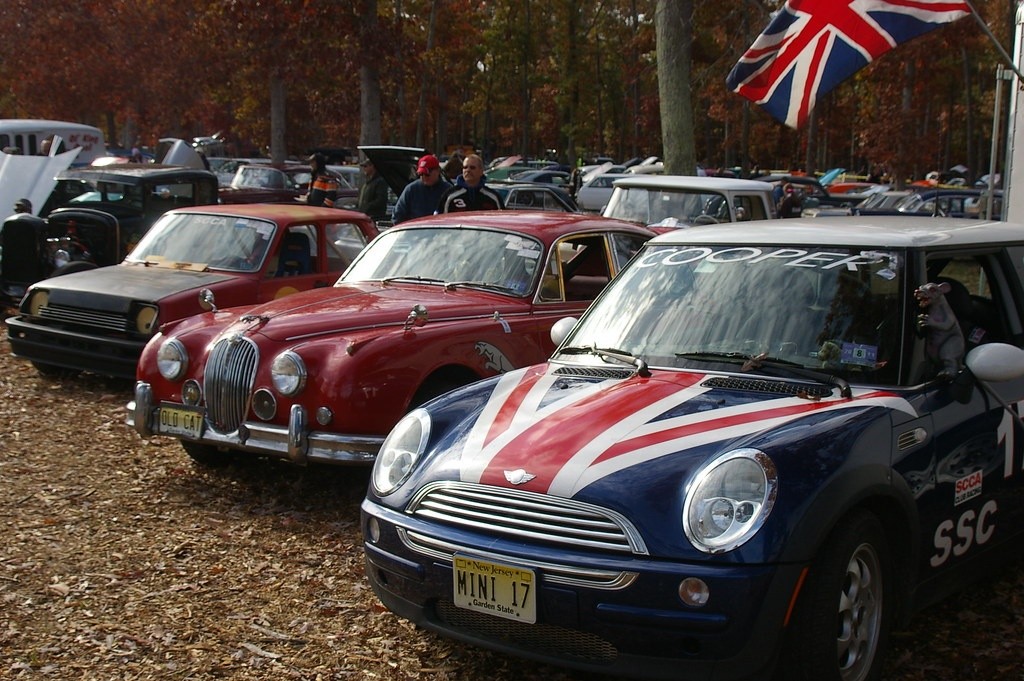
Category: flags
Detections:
[{"x1": 725, "y1": 0, "x2": 975, "y2": 132}]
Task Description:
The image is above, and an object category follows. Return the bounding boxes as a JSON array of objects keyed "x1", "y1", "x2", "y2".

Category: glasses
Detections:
[
  {"x1": 463, "y1": 165, "x2": 475, "y2": 170},
  {"x1": 420, "y1": 173, "x2": 430, "y2": 177}
]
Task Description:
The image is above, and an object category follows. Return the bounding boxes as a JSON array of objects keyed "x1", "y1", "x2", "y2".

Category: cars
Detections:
[
  {"x1": 129, "y1": 209, "x2": 657, "y2": 473},
  {"x1": 3, "y1": 203, "x2": 385, "y2": 379},
  {"x1": 0, "y1": 164, "x2": 220, "y2": 309},
  {"x1": 96, "y1": 132, "x2": 1006, "y2": 274},
  {"x1": 359, "y1": 220, "x2": 1023, "y2": 679}
]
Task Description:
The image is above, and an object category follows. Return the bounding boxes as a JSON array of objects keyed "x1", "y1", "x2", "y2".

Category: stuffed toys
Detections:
[{"x1": 818, "y1": 340, "x2": 864, "y2": 372}]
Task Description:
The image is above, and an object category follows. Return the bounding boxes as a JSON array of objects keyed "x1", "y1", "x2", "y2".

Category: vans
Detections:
[{"x1": 0, "y1": 120, "x2": 105, "y2": 170}]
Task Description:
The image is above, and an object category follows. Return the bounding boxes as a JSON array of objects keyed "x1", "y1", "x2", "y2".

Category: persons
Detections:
[
  {"x1": 194, "y1": 146, "x2": 211, "y2": 171},
  {"x1": 36, "y1": 140, "x2": 52, "y2": 156},
  {"x1": 857, "y1": 160, "x2": 896, "y2": 186},
  {"x1": 542, "y1": 148, "x2": 582, "y2": 168},
  {"x1": 132, "y1": 147, "x2": 148, "y2": 164},
  {"x1": 0, "y1": 199, "x2": 44, "y2": 247},
  {"x1": 712, "y1": 160, "x2": 772, "y2": 179},
  {"x1": 304, "y1": 150, "x2": 507, "y2": 254},
  {"x1": 774, "y1": 175, "x2": 802, "y2": 219}
]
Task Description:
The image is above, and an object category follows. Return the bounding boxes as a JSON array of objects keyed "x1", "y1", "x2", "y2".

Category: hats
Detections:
[
  {"x1": 360, "y1": 159, "x2": 371, "y2": 166},
  {"x1": 305, "y1": 154, "x2": 325, "y2": 162},
  {"x1": 416, "y1": 155, "x2": 438, "y2": 172}
]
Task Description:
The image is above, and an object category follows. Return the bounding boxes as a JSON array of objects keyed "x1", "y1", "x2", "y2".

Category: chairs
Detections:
[
  {"x1": 734, "y1": 267, "x2": 821, "y2": 356},
  {"x1": 276, "y1": 233, "x2": 311, "y2": 278},
  {"x1": 935, "y1": 277, "x2": 1001, "y2": 366}
]
